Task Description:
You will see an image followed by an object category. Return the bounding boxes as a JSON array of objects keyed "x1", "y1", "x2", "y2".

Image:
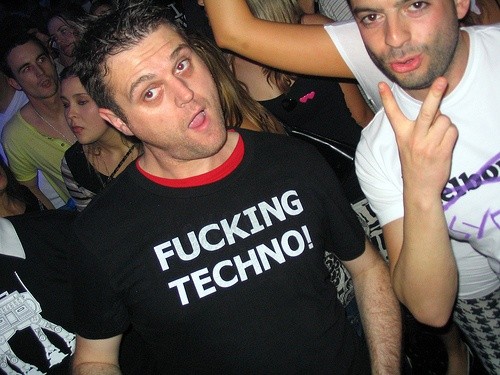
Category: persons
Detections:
[{"x1": 1, "y1": 0, "x2": 500, "y2": 375}]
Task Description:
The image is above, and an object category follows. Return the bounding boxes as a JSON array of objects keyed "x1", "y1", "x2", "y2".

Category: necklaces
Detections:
[
  {"x1": 31, "y1": 104, "x2": 80, "y2": 151},
  {"x1": 100, "y1": 146, "x2": 132, "y2": 182}
]
